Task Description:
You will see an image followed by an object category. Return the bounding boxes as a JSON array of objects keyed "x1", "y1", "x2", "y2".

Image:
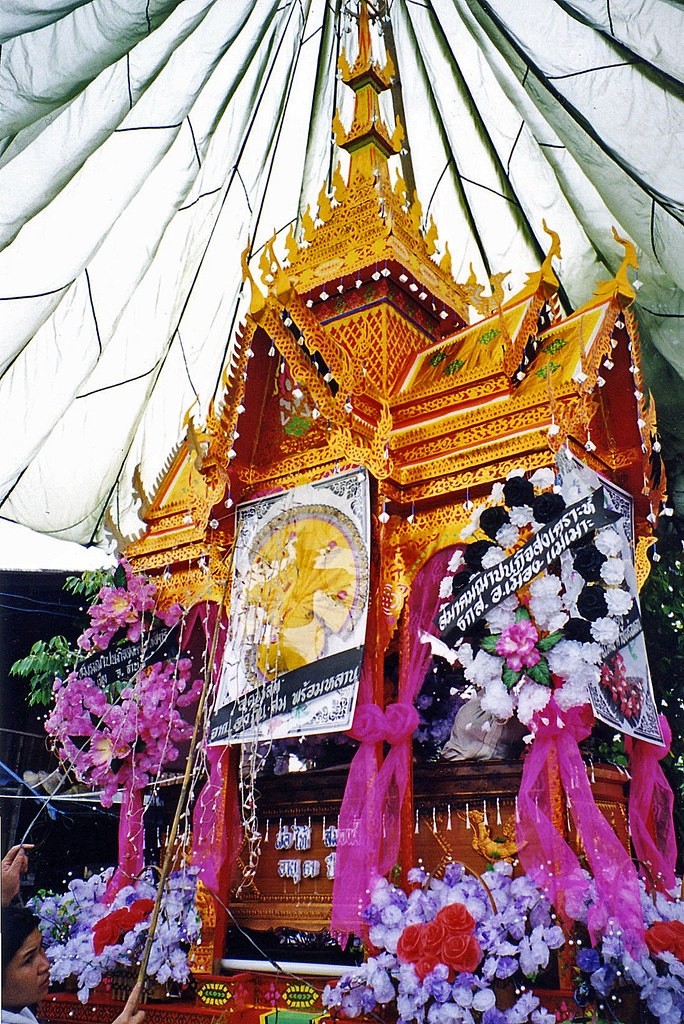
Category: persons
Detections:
[{"x1": 1, "y1": 843, "x2": 145, "y2": 1024}]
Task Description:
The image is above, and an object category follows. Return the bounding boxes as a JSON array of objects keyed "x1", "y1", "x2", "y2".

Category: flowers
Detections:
[{"x1": 24, "y1": 467, "x2": 684, "y2": 1024}]
